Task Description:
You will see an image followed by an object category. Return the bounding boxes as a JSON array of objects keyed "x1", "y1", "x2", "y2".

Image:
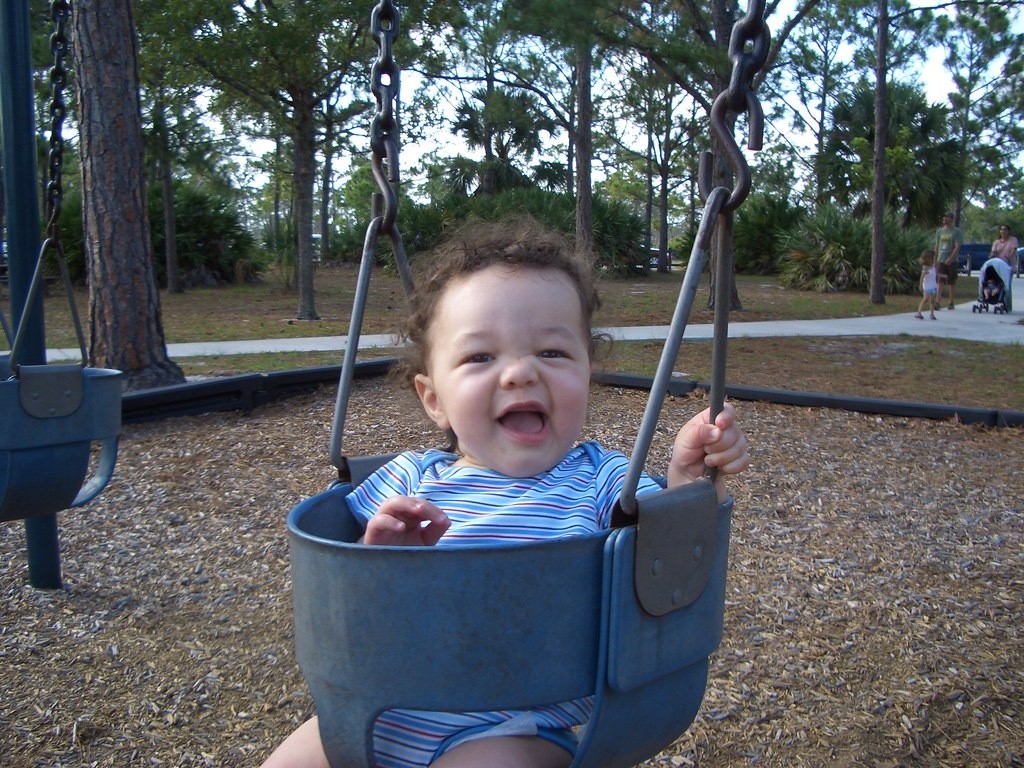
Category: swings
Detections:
[
  {"x1": 283, "y1": 1, "x2": 774, "y2": 766},
  {"x1": 1, "y1": 0, "x2": 127, "y2": 520}
]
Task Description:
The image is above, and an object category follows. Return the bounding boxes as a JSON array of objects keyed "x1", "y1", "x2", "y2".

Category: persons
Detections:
[
  {"x1": 988, "y1": 224, "x2": 1019, "y2": 311},
  {"x1": 914, "y1": 249, "x2": 948, "y2": 320},
  {"x1": 984, "y1": 279, "x2": 1000, "y2": 300},
  {"x1": 259, "y1": 214, "x2": 751, "y2": 768},
  {"x1": 932, "y1": 211, "x2": 963, "y2": 310}
]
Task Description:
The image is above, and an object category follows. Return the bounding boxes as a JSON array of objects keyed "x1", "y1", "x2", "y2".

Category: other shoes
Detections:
[
  {"x1": 914, "y1": 312, "x2": 924, "y2": 319},
  {"x1": 930, "y1": 314, "x2": 936, "y2": 320},
  {"x1": 935, "y1": 302, "x2": 940, "y2": 310},
  {"x1": 947, "y1": 303, "x2": 954, "y2": 309}
]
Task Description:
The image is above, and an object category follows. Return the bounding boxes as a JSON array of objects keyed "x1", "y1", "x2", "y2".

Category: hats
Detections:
[{"x1": 943, "y1": 211, "x2": 954, "y2": 218}]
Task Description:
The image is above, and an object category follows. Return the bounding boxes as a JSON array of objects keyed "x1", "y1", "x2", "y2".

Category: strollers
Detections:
[{"x1": 971, "y1": 258, "x2": 1014, "y2": 315}]
